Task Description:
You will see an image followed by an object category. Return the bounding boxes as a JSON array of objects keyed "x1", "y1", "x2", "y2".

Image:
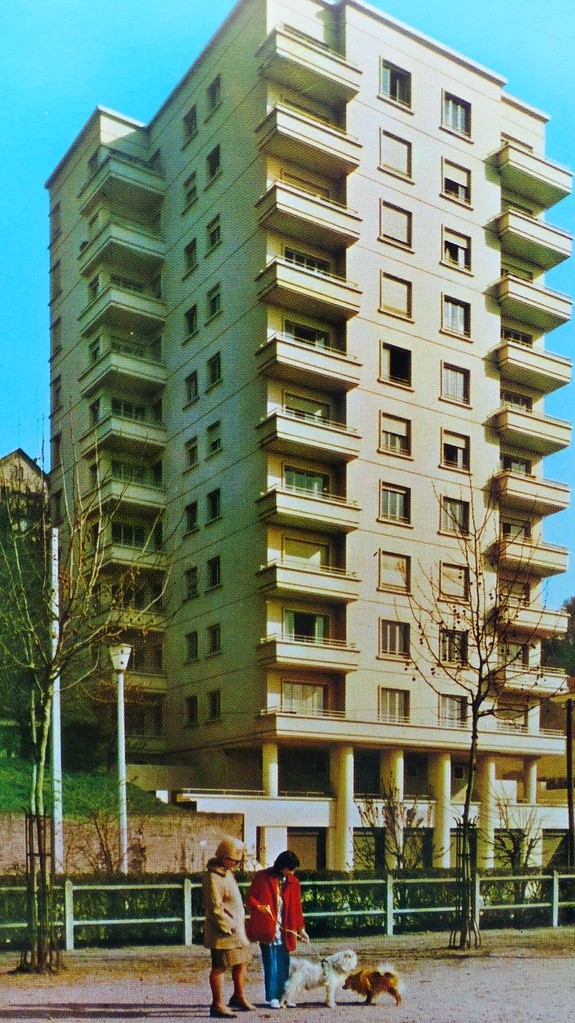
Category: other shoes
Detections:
[
  {"x1": 210, "y1": 1006, "x2": 236, "y2": 1018},
  {"x1": 270, "y1": 998, "x2": 280, "y2": 1008},
  {"x1": 230, "y1": 995, "x2": 256, "y2": 1011},
  {"x1": 285, "y1": 998, "x2": 296, "y2": 1008}
]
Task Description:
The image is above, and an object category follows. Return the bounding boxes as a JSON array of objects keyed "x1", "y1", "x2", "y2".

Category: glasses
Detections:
[{"x1": 226, "y1": 856, "x2": 240, "y2": 865}]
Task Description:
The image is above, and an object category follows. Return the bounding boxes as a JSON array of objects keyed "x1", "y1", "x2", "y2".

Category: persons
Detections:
[
  {"x1": 244, "y1": 850, "x2": 310, "y2": 1008},
  {"x1": 202, "y1": 836, "x2": 254, "y2": 1019}
]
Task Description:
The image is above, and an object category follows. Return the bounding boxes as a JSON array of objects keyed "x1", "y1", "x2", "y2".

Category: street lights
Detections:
[{"x1": 107, "y1": 642, "x2": 133, "y2": 874}]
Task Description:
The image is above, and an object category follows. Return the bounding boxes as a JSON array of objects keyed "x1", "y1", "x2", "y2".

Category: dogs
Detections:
[
  {"x1": 342, "y1": 963, "x2": 404, "y2": 1007},
  {"x1": 279, "y1": 949, "x2": 358, "y2": 1008}
]
